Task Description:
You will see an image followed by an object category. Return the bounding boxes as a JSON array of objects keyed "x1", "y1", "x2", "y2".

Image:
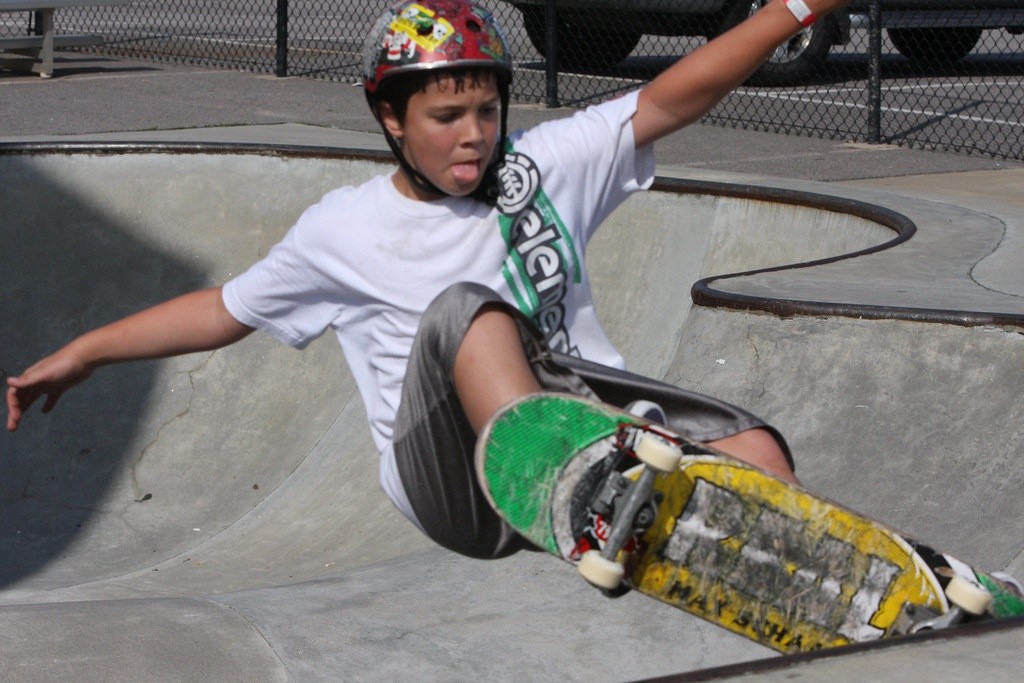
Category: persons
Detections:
[{"x1": 6, "y1": 0, "x2": 835, "y2": 561}]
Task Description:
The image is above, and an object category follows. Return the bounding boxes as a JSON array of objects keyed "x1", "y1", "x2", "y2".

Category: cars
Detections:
[{"x1": 507, "y1": 0, "x2": 1024, "y2": 87}]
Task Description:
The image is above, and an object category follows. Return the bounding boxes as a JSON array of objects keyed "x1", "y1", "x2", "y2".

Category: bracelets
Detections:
[{"x1": 782, "y1": 0, "x2": 818, "y2": 31}]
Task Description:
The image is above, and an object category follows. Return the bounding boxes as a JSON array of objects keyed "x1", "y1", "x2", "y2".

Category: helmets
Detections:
[{"x1": 362, "y1": 0, "x2": 512, "y2": 119}]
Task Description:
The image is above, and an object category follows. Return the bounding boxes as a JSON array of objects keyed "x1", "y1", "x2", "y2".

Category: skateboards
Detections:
[{"x1": 473, "y1": 389, "x2": 1024, "y2": 656}]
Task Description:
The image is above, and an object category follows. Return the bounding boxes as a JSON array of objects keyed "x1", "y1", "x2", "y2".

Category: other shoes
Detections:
[
  {"x1": 597, "y1": 397, "x2": 668, "y2": 598},
  {"x1": 990, "y1": 569, "x2": 1024, "y2": 595}
]
